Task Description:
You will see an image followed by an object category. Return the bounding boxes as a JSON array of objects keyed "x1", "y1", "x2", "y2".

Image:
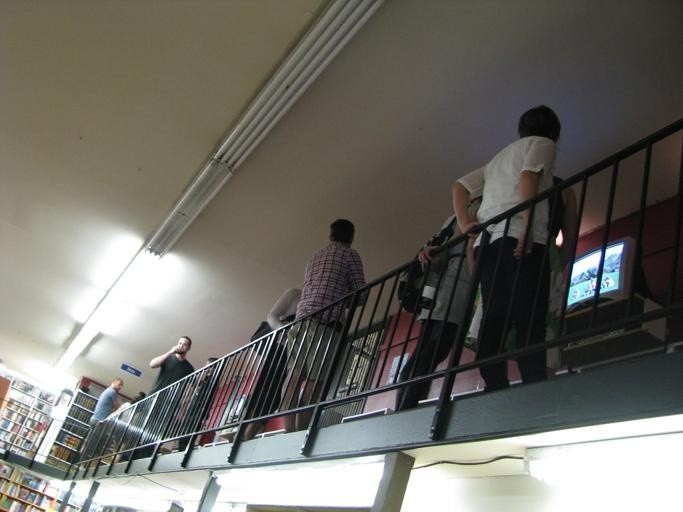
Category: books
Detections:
[{"x1": 0, "y1": 393, "x2": 111, "y2": 512}]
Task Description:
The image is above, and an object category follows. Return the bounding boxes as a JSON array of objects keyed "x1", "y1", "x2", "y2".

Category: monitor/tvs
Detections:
[{"x1": 566, "y1": 235, "x2": 653, "y2": 312}]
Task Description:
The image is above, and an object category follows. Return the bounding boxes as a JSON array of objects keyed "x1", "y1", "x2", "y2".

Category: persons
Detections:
[
  {"x1": 450, "y1": 102, "x2": 562, "y2": 390},
  {"x1": 171, "y1": 357, "x2": 224, "y2": 454},
  {"x1": 98, "y1": 391, "x2": 146, "y2": 463},
  {"x1": 417, "y1": 173, "x2": 586, "y2": 380},
  {"x1": 79, "y1": 377, "x2": 121, "y2": 469},
  {"x1": 278, "y1": 216, "x2": 369, "y2": 433},
  {"x1": 391, "y1": 191, "x2": 482, "y2": 412},
  {"x1": 148, "y1": 335, "x2": 195, "y2": 455},
  {"x1": 238, "y1": 284, "x2": 303, "y2": 442}
]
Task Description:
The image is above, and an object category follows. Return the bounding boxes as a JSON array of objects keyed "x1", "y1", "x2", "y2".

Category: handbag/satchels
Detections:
[
  {"x1": 397, "y1": 229, "x2": 453, "y2": 312},
  {"x1": 250, "y1": 321, "x2": 273, "y2": 358}
]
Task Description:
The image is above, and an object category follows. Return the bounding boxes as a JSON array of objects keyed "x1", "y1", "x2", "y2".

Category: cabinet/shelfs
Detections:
[{"x1": 0, "y1": 374, "x2": 135, "y2": 510}]
